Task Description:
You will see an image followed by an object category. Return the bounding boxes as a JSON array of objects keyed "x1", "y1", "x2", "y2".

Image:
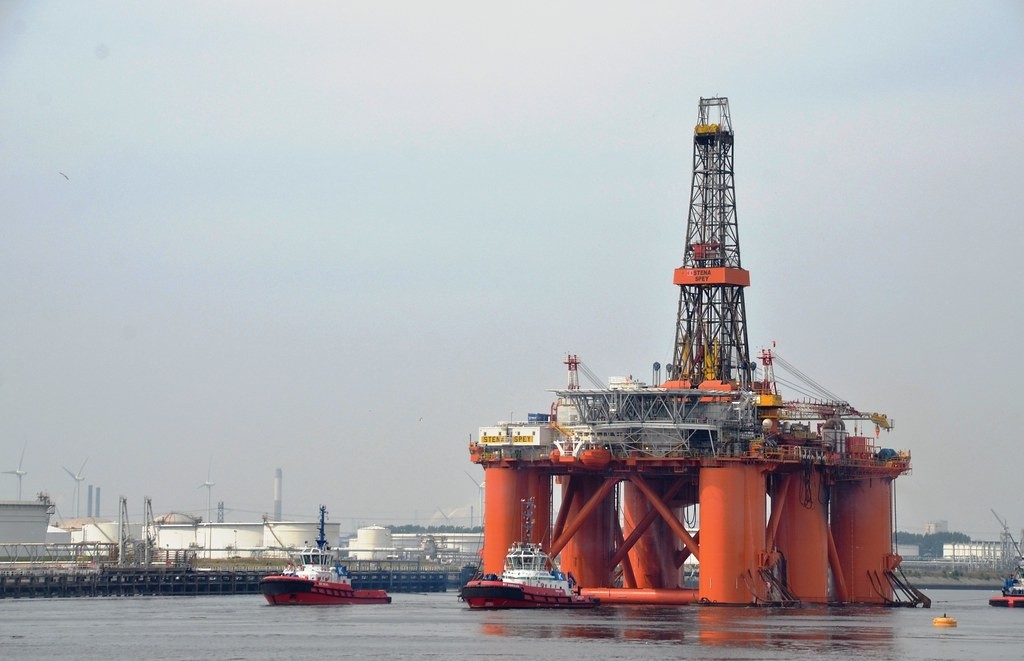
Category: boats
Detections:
[
  {"x1": 460, "y1": 579, "x2": 599, "y2": 609},
  {"x1": 988, "y1": 587, "x2": 1024, "y2": 607},
  {"x1": 259, "y1": 576, "x2": 393, "y2": 605}
]
